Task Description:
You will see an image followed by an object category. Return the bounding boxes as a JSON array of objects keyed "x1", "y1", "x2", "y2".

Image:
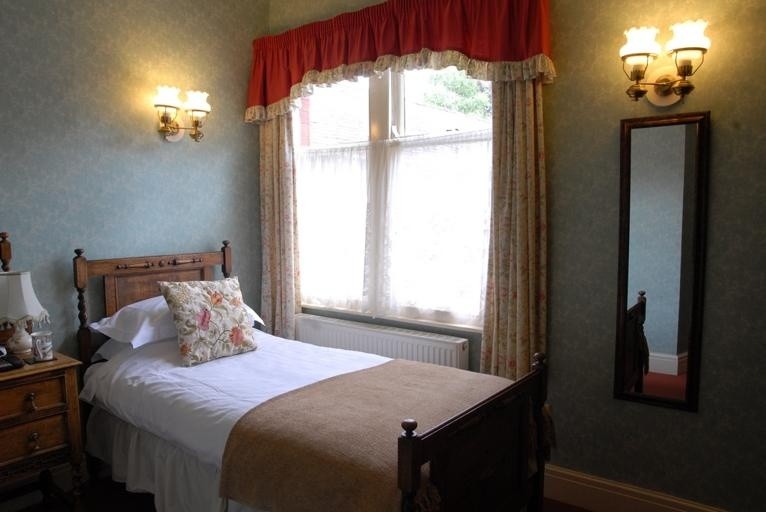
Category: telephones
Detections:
[{"x1": 0, "y1": 346, "x2": 25, "y2": 373}]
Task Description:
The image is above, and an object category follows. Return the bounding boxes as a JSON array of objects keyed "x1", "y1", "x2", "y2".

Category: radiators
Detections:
[{"x1": 296, "y1": 312, "x2": 470, "y2": 370}]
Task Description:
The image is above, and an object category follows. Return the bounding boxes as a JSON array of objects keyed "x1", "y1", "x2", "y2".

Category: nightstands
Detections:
[{"x1": 1, "y1": 349, "x2": 87, "y2": 511}]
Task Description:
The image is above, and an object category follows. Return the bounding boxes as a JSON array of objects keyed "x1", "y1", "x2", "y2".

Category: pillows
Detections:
[{"x1": 88, "y1": 276, "x2": 266, "y2": 367}]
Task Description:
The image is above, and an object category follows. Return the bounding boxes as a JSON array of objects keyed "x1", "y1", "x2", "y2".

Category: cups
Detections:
[{"x1": 29, "y1": 331, "x2": 53, "y2": 361}]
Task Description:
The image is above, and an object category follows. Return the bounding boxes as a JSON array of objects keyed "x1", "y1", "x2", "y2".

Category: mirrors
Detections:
[{"x1": 614, "y1": 111, "x2": 710, "y2": 413}]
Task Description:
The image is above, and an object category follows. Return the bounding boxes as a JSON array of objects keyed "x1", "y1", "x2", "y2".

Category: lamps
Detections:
[
  {"x1": 619, "y1": 18, "x2": 712, "y2": 109},
  {"x1": 153, "y1": 86, "x2": 212, "y2": 144},
  {"x1": 0, "y1": 231, "x2": 51, "y2": 360}
]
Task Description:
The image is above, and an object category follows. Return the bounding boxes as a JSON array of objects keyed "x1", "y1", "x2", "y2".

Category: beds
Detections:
[{"x1": 72, "y1": 239, "x2": 548, "y2": 512}]
